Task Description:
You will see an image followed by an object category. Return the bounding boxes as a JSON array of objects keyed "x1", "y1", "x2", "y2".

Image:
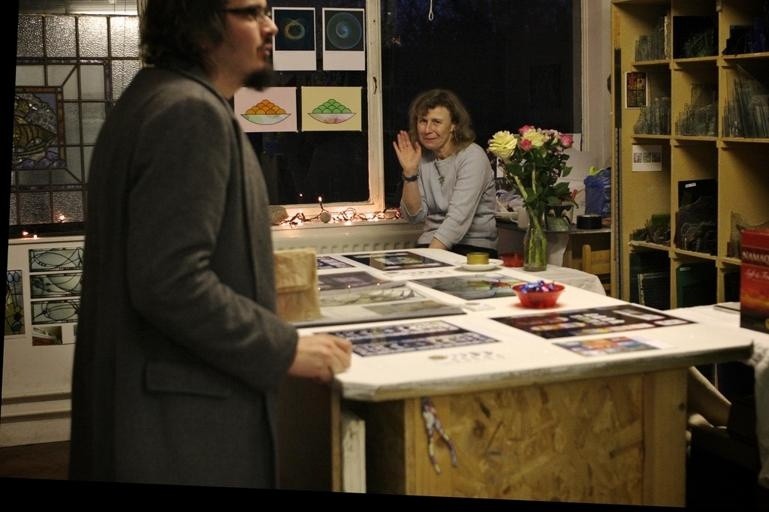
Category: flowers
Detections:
[{"x1": 486, "y1": 124, "x2": 581, "y2": 223}]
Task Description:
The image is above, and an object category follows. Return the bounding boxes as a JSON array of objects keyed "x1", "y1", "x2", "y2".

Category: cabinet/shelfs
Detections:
[{"x1": 612, "y1": 0, "x2": 769, "y2": 310}]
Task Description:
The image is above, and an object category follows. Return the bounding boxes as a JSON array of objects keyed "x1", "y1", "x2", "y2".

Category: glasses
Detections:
[{"x1": 225, "y1": 4, "x2": 271, "y2": 21}]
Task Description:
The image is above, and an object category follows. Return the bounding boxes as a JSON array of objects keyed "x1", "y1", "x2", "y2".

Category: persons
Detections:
[
  {"x1": 69, "y1": 0, "x2": 352, "y2": 490},
  {"x1": 392, "y1": 88, "x2": 500, "y2": 258}
]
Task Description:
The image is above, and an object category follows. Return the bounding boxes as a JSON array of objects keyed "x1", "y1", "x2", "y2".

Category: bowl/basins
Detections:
[{"x1": 511, "y1": 283, "x2": 565, "y2": 309}]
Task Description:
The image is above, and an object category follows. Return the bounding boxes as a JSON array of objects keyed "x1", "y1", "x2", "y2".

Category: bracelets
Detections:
[{"x1": 401, "y1": 172, "x2": 419, "y2": 182}]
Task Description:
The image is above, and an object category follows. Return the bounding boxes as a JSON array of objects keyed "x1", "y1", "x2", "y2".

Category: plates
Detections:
[{"x1": 462, "y1": 259, "x2": 504, "y2": 271}]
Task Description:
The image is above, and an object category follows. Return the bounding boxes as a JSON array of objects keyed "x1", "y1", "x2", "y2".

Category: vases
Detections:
[{"x1": 522, "y1": 224, "x2": 549, "y2": 271}]
